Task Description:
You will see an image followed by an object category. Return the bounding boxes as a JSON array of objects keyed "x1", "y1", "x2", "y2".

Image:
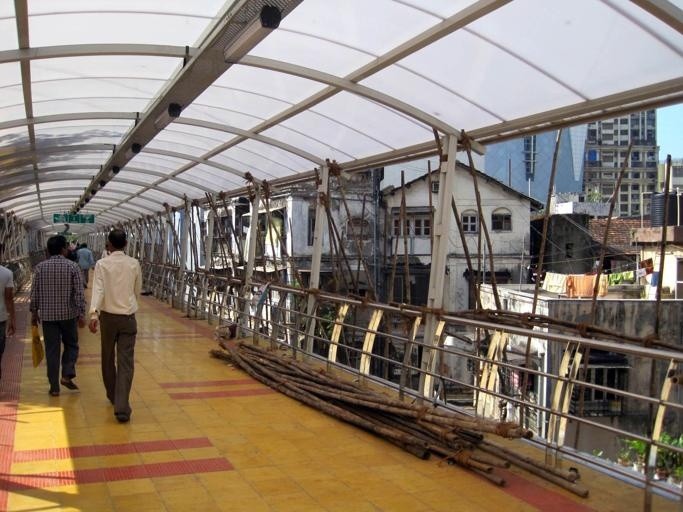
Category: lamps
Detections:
[
  {"x1": 154, "y1": 103, "x2": 180, "y2": 129},
  {"x1": 222, "y1": 4, "x2": 282, "y2": 64}
]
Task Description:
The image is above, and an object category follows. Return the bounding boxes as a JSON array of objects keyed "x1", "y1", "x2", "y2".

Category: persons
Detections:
[
  {"x1": 88, "y1": 230, "x2": 142, "y2": 421},
  {"x1": 0, "y1": 244, "x2": 16, "y2": 394},
  {"x1": 101, "y1": 243, "x2": 110, "y2": 259},
  {"x1": 28, "y1": 236, "x2": 87, "y2": 396},
  {"x1": 67, "y1": 239, "x2": 93, "y2": 290}
]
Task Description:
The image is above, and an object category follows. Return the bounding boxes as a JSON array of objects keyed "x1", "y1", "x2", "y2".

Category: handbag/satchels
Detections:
[{"x1": 31, "y1": 326, "x2": 44, "y2": 370}]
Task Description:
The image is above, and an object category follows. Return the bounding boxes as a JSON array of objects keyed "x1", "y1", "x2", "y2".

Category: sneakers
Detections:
[{"x1": 113, "y1": 411, "x2": 130, "y2": 424}]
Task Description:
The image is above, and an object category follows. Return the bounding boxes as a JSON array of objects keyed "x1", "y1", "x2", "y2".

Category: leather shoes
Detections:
[
  {"x1": 60, "y1": 378, "x2": 79, "y2": 390},
  {"x1": 48, "y1": 390, "x2": 60, "y2": 397}
]
